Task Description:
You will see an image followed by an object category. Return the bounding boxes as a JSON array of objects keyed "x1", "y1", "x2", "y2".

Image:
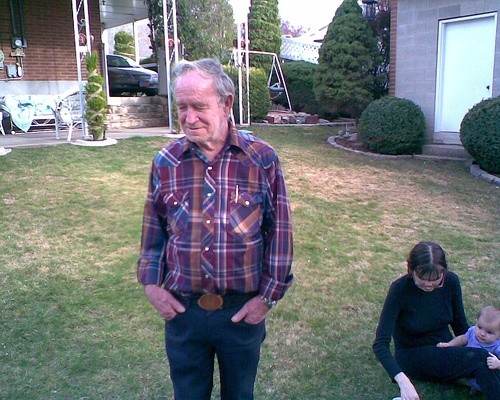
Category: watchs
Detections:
[{"x1": 258, "y1": 294, "x2": 278, "y2": 309}]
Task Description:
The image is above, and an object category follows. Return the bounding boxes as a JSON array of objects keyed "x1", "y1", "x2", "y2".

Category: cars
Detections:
[{"x1": 106, "y1": 54, "x2": 159, "y2": 96}]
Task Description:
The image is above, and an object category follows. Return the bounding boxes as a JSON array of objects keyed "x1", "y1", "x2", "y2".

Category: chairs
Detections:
[{"x1": 48, "y1": 89, "x2": 88, "y2": 143}]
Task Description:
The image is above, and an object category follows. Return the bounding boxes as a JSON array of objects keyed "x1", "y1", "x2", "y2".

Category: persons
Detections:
[
  {"x1": 371, "y1": 240, "x2": 500, "y2": 400},
  {"x1": 136, "y1": 57, "x2": 295, "y2": 400}
]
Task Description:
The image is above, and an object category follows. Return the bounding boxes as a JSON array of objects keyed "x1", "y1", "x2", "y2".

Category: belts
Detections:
[{"x1": 171, "y1": 291, "x2": 260, "y2": 313}]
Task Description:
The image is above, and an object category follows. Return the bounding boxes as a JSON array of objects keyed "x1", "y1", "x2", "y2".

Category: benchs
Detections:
[{"x1": 2, "y1": 93, "x2": 82, "y2": 134}]
